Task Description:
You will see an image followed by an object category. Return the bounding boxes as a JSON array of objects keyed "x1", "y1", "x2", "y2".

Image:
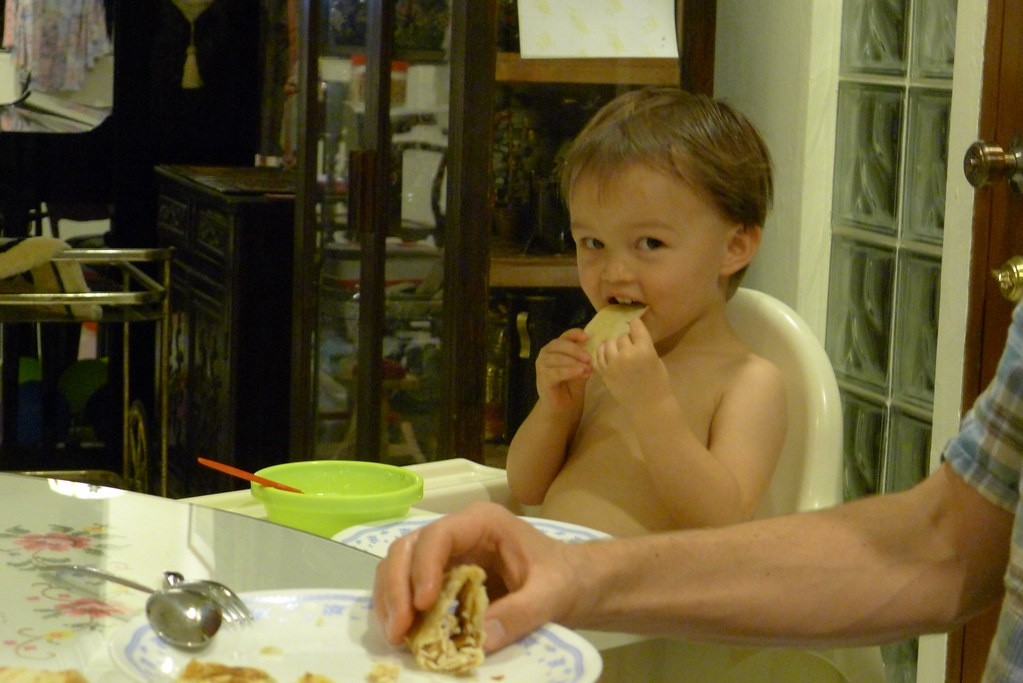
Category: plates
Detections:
[
  {"x1": 109, "y1": 588, "x2": 603, "y2": 682},
  {"x1": 331, "y1": 515, "x2": 615, "y2": 558}
]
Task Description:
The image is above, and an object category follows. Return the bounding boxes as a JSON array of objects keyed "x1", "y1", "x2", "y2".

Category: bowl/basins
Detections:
[{"x1": 251, "y1": 460, "x2": 424, "y2": 540}]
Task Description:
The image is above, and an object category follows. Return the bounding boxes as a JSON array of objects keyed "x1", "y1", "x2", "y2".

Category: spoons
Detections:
[{"x1": 41, "y1": 564, "x2": 223, "y2": 649}]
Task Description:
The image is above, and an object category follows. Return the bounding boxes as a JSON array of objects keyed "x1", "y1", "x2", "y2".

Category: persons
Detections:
[
  {"x1": 506, "y1": 88, "x2": 787, "y2": 536},
  {"x1": 374, "y1": 299, "x2": 1023, "y2": 682}
]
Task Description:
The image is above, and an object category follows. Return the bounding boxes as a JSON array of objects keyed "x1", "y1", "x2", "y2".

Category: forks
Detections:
[{"x1": 164, "y1": 572, "x2": 252, "y2": 624}]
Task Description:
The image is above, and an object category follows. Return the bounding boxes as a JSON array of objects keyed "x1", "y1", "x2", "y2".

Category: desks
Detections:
[{"x1": 0, "y1": 471, "x2": 844, "y2": 683}]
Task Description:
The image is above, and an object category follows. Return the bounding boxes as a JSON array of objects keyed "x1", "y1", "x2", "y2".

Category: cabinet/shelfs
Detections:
[{"x1": 156, "y1": 163, "x2": 297, "y2": 495}]
